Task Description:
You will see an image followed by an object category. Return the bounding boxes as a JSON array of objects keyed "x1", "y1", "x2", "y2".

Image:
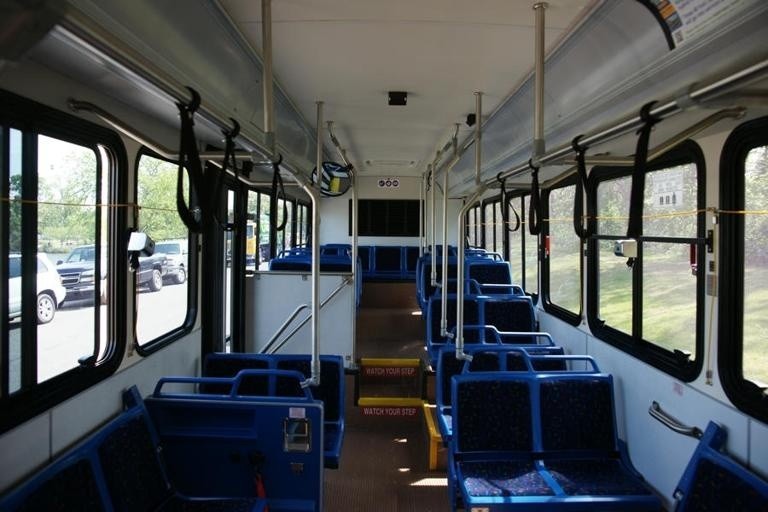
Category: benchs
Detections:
[
  {"x1": 269, "y1": 243, "x2": 542, "y2": 371},
  {"x1": 436, "y1": 325, "x2": 768, "y2": 512},
  {"x1": 1, "y1": 352, "x2": 345, "y2": 510}
]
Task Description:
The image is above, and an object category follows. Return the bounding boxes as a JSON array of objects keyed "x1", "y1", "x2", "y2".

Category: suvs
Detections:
[{"x1": 57, "y1": 236, "x2": 188, "y2": 307}]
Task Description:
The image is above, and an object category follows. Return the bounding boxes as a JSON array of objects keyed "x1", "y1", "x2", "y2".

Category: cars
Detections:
[{"x1": 7, "y1": 246, "x2": 69, "y2": 325}]
